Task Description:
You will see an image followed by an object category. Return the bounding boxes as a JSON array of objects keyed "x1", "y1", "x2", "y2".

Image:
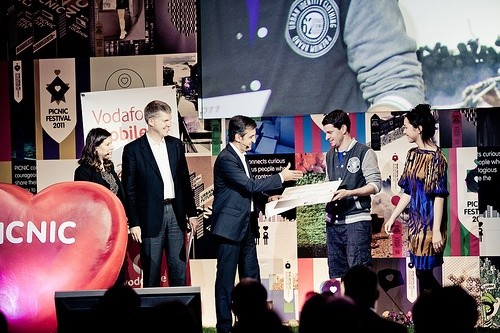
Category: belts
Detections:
[{"x1": 164, "y1": 199, "x2": 175, "y2": 205}]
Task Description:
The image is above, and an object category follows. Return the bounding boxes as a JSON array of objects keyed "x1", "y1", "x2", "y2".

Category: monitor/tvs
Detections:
[{"x1": 54, "y1": 285, "x2": 203, "y2": 333}]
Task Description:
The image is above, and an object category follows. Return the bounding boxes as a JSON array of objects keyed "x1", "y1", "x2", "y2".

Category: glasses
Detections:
[{"x1": 402, "y1": 125, "x2": 411, "y2": 129}]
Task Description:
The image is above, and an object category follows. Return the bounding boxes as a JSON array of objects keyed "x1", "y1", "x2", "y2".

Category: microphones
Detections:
[
  {"x1": 98, "y1": 153, "x2": 109, "y2": 156},
  {"x1": 234, "y1": 140, "x2": 249, "y2": 148}
]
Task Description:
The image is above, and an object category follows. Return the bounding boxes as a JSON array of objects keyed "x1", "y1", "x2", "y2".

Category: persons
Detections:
[
  {"x1": 322, "y1": 110, "x2": 382, "y2": 281},
  {"x1": 198, "y1": 0, "x2": 425, "y2": 121},
  {"x1": 122, "y1": 100, "x2": 199, "y2": 288},
  {"x1": 74, "y1": 127, "x2": 122, "y2": 288},
  {"x1": 385, "y1": 103, "x2": 450, "y2": 298},
  {"x1": 57, "y1": 266, "x2": 500, "y2": 333},
  {"x1": 210, "y1": 116, "x2": 303, "y2": 333}
]
